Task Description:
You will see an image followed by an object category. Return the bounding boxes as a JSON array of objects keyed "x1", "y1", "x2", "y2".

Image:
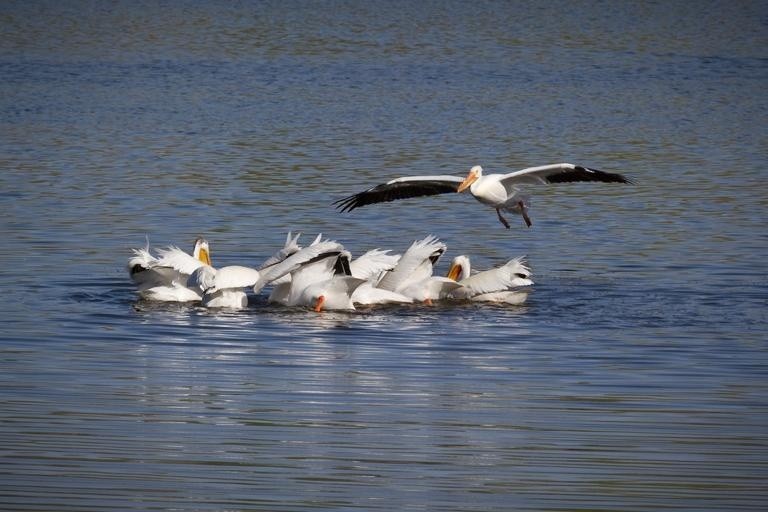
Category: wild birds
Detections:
[
  {"x1": 127, "y1": 230, "x2": 535, "y2": 312},
  {"x1": 328, "y1": 163, "x2": 640, "y2": 229}
]
